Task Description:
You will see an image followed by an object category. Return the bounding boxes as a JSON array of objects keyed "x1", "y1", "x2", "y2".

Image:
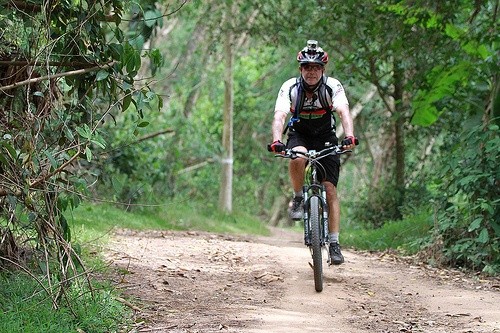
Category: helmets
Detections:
[{"x1": 297, "y1": 46, "x2": 328, "y2": 65}]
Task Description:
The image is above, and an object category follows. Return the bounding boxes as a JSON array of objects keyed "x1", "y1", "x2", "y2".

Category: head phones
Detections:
[{"x1": 299, "y1": 76, "x2": 322, "y2": 93}]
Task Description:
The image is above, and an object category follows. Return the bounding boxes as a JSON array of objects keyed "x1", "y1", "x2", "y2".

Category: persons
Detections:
[{"x1": 271, "y1": 40, "x2": 354, "y2": 265}]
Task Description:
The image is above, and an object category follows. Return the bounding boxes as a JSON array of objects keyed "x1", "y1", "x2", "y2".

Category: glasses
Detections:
[{"x1": 301, "y1": 65, "x2": 322, "y2": 72}]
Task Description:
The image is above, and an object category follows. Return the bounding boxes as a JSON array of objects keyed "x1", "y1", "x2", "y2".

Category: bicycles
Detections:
[{"x1": 268, "y1": 137, "x2": 359, "y2": 292}]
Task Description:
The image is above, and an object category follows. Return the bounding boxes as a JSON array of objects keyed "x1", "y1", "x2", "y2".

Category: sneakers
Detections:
[
  {"x1": 329, "y1": 242, "x2": 343, "y2": 264},
  {"x1": 291, "y1": 195, "x2": 304, "y2": 219}
]
